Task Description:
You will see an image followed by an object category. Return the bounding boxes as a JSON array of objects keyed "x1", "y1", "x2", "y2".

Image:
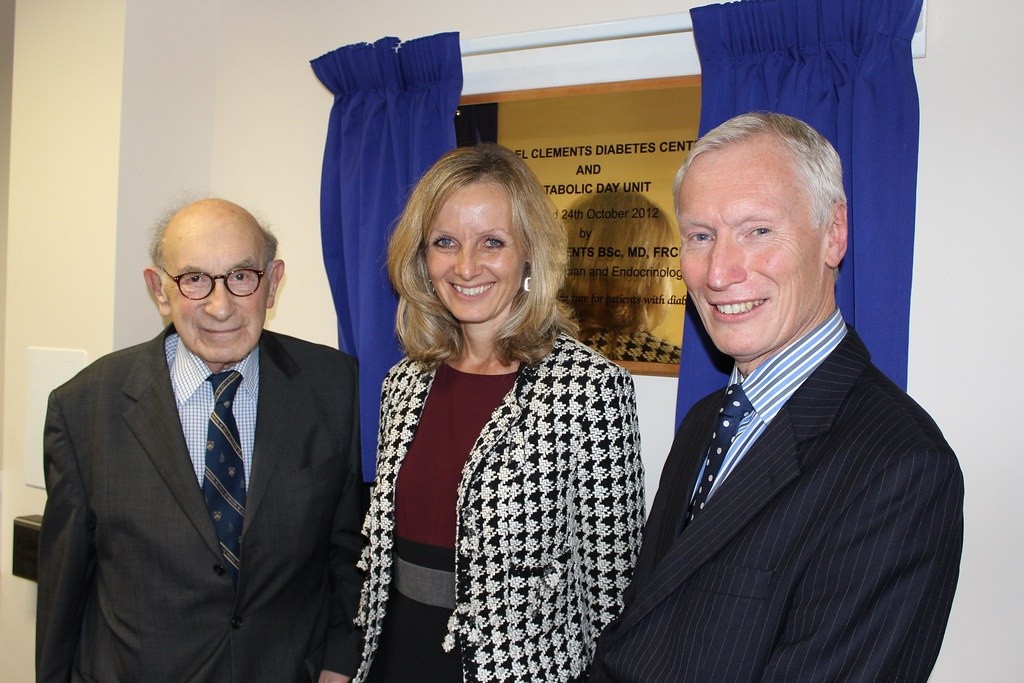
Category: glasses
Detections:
[{"x1": 157, "y1": 264, "x2": 268, "y2": 301}]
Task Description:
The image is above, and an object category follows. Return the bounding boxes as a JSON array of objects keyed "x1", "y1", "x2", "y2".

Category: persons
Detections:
[
  {"x1": 36, "y1": 199, "x2": 362, "y2": 683},
  {"x1": 352, "y1": 146, "x2": 645, "y2": 683},
  {"x1": 579, "y1": 111, "x2": 964, "y2": 683},
  {"x1": 556, "y1": 191, "x2": 682, "y2": 364}
]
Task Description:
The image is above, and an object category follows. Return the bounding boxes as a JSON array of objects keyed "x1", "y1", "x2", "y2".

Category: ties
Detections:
[
  {"x1": 682, "y1": 384, "x2": 755, "y2": 533},
  {"x1": 202, "y1": 371, "x2": 246, "y2": 581}
]
